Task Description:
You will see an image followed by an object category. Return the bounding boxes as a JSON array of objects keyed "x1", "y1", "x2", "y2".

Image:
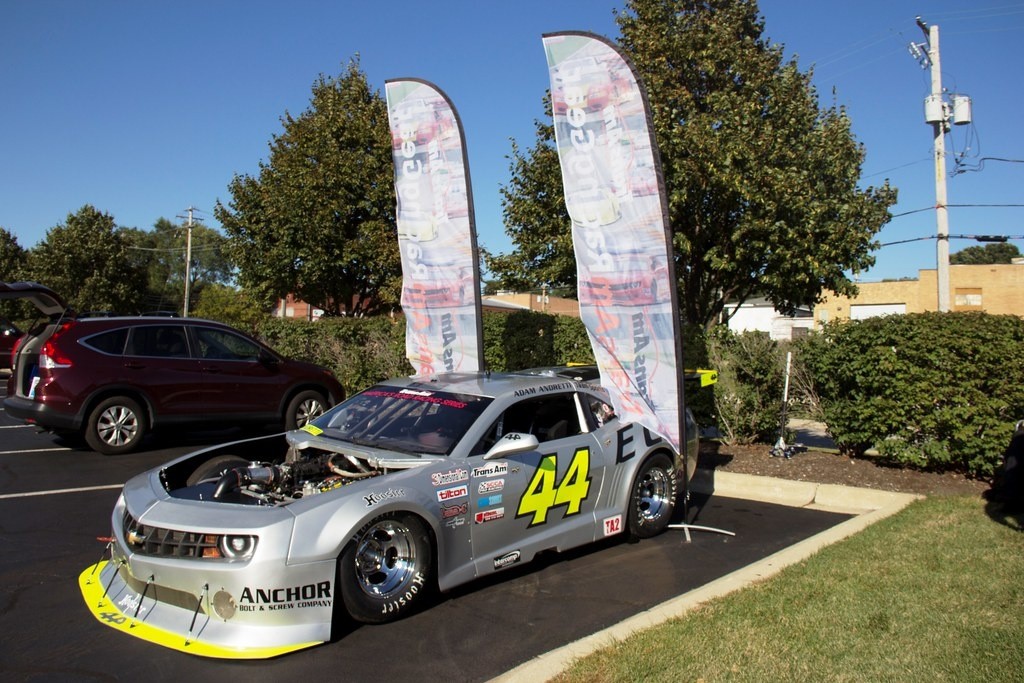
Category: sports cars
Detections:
[{"x1": 77, "y1": 363, "x2": 700, "y2": 662}]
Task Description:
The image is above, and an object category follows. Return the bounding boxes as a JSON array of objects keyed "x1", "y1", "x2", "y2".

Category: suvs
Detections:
[{"x1": 0, "y1": 279, "x2": 350, "y2": 448}]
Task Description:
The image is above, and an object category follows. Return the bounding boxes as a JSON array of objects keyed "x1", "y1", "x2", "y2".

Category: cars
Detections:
[{"x1": 998, "y1": 419, "x2": 1024, "y2": 529}]
